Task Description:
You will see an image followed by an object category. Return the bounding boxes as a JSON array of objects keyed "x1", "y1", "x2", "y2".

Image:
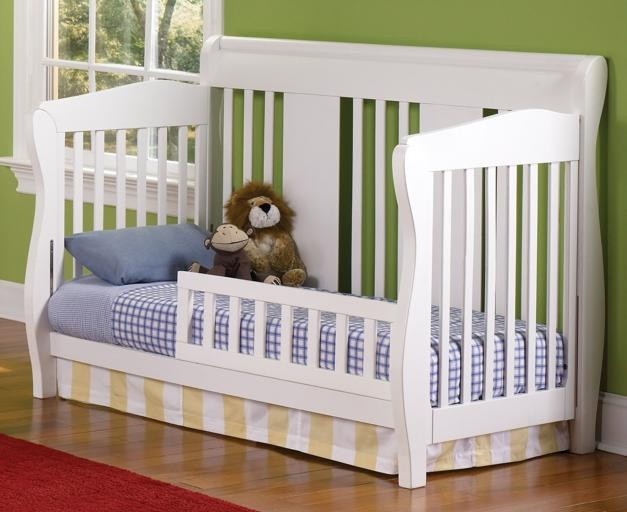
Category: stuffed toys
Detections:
[
  {"x1": 190, "y1": 225, "x2": 253, "y2": 280},
  {"x1": 225, "y1": 180, "x2": 307, "y2": 291}
]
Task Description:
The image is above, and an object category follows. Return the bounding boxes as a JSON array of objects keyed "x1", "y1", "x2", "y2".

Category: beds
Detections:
[{"x1": 23, "y1": 33, "x2": 608, "y2": 489}]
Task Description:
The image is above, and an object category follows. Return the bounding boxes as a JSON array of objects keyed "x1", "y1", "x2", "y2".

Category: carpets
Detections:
[{"x1": 0, "y1": 434, "x2": 259, "y2": 511}]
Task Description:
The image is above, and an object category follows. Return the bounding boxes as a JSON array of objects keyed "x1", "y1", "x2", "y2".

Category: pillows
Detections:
[{"x1": 64, "y1": 223, "x2": 215, "y2": 286}]
